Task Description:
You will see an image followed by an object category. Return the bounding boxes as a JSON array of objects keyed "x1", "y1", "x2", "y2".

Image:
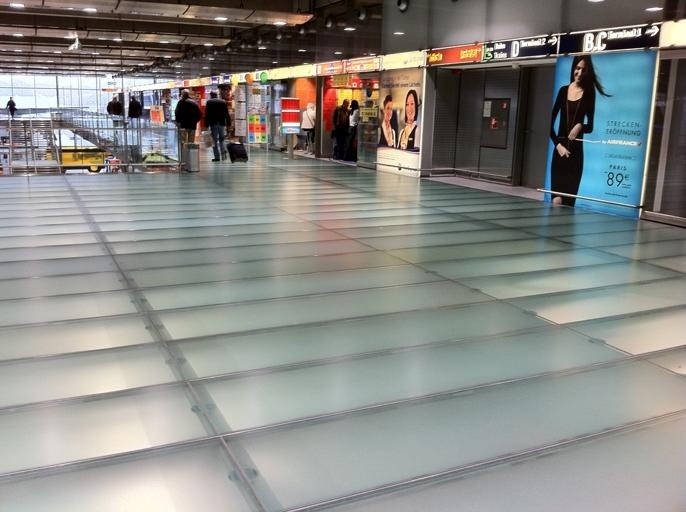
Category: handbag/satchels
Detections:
[{"x1": 201, "y1": 130, "x2": 212, "y2": 148}]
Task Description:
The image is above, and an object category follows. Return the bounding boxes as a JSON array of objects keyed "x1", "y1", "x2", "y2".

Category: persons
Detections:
[
  {"x1": 396, "y1": 90, "x2": 421, "y2": 150},
  {"x1": 301, "y1": 102, "x2": 316, "y2": 146},
  {"x1": 331, "y1": 99, "x2": 351, "y2": 160},
  {"x1": 342, "y1": 100, "x2": 360, "y2": 160},
  {"x1": 378, "y1": 95, "x2": 397, "y2": 148},
  {"x1": 175, "y1": 89, "x2": 202, "y2": 142},
  {"x1": 128, "y1": 96, "x2": 143, "y2": 118},
  {"x1": 550, "y1": 54, "x2": 614, "y2": 207},
  {"x1": 204, "y1": 92, "x2": 232, "y2": 161},
  {"x1": 6, "y1": 97, "x2": 18, "y2": 119},
  {"x1": 107, "y1": 97, "x2": 123, "y2": 127}
]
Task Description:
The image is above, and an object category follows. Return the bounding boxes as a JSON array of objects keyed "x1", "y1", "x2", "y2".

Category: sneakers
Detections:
[{"x1": 212, "y1": 158, "x2": 219, "y2": 161}]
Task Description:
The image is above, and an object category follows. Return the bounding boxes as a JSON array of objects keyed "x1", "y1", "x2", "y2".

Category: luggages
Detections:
[{"x1": 226, "y1": 130, "x2": 248, "y2": 163}]
